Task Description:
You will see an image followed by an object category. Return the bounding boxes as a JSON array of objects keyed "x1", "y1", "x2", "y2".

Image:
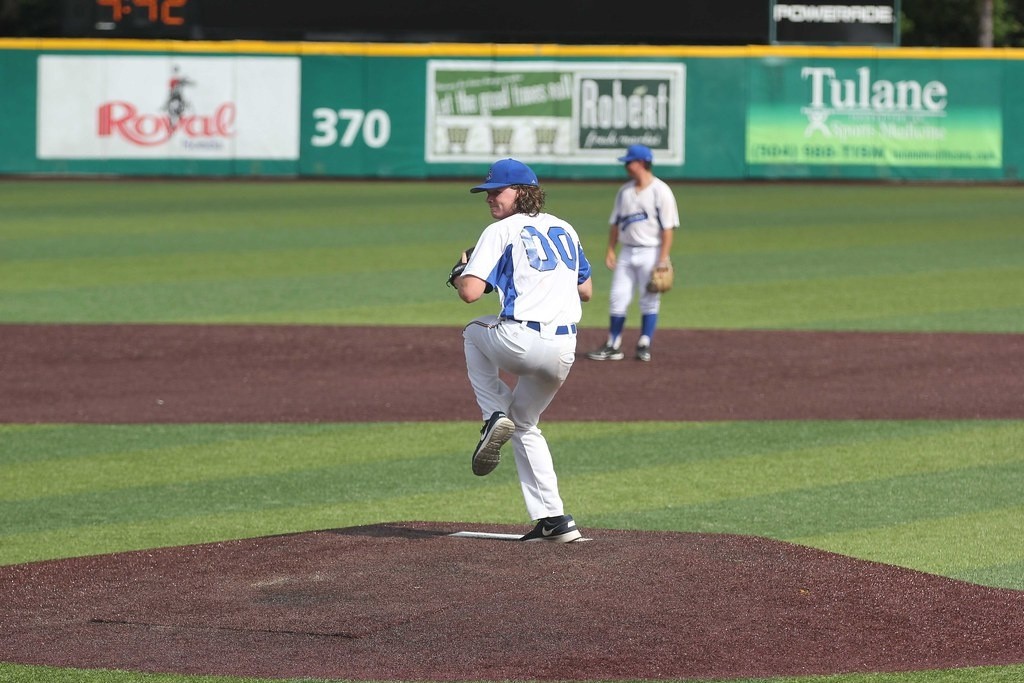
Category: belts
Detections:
[{"x1": 512, "y1": 318, "x2": 578, "y2": 335}]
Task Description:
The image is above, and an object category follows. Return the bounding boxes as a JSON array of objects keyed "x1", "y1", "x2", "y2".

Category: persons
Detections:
[
  {"x1": 581, "y1": 144, "x2": 678, "y2": 364},
  {"x1": 446, "y1": 157, "x2": 591, "y2": 544}
]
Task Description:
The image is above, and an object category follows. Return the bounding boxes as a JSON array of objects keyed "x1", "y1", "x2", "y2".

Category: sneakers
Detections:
[
  {"x1": 520, "y1": 514, "x2": 582, "y2": 544},
  {"x1": 472, "y1": 411, "x2": 516, "y2": 476},
  {"x1": 586, "y1": 342, "x2": 625, "y2": 360},
  {"x1": 635, "y1": 345, "x2": 651, "y2": 362}
]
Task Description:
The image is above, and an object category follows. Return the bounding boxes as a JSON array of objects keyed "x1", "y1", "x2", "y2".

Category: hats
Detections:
[
  {"x1": 617, "y1": 144, "x2": 652, "y2": 161},
  {"x1": 470, "y1": 158, "x2": 539, "y2": 194}
]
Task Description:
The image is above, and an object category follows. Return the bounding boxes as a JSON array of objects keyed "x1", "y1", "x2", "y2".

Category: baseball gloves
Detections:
[
  {"x1": 446, "y1": 248, "x2": 477, "y2": 287},
  {"x1": 647, "y1": 262, "x2": 674, "y2": 292}
]
